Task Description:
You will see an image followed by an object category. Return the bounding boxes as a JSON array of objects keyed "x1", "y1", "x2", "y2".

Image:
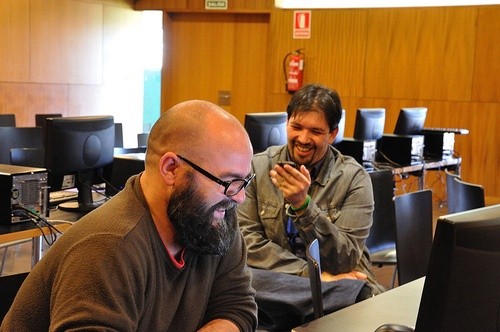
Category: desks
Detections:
[
  {"x1": 279, "y1": 274, "x2": 425, "y2": 332},
  {"x1": 370, "y1": 156, "x2": 464, "y2": 191},
  {"x1": 0, "y1": 191, "x2": 113, "y2": 278},
  {"x1": 105, "y1": 151, "x2": 147, "y2": 200}
]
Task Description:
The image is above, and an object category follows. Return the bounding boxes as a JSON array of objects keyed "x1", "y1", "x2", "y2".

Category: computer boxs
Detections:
[
  {"x1": 336, "y1": 133, "x2": 457, "y2": 173},
  {"x1": 105, "y1": 153, "x2": 148, "y2": 197},
  {"x1": 0, "y1": 163, "x2": 51, "y2": 226}
]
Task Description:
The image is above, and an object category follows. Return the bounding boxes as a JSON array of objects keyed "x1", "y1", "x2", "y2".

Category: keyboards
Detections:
[{"x1": 422, "y1": 128, "x2": 469, "y2": 134}]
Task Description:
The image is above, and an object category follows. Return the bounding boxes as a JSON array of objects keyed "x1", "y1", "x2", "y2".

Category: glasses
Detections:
[{"x1": 175, "y1": 154, "x2": 256, "y2": 196}]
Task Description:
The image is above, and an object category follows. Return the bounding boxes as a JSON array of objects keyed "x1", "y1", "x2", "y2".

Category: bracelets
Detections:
[{"x1": 290, "y1": 194, "x2": 310, "y2": 212}]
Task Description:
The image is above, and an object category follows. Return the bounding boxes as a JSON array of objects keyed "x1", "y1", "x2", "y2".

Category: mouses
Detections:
[{"x1": 374, "y1": 323, "x2": 414, "y2": 332}]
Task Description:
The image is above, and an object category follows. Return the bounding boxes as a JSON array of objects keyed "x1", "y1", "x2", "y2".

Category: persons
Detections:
[
  {"x1": 1, "y1": 99, "x2": 259, "y2": 332},
  {"x1": 238, "y1": 84, "x2": 386, "y2": 329}
]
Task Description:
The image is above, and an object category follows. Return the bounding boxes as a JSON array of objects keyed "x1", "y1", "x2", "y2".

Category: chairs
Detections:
[{"x1": 365, "y1": 169, "x2": 491, "y2": 286}]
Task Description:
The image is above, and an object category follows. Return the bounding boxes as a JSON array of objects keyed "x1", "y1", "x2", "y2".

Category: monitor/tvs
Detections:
[
  {"x1": 244, "y1": 112, "x2": 288, "y2": 155},
  {"x1": 44, "y1": 115, "x2": 115, "y2": 212},
  {"x1": 354, "y1": 108, "x2": 386, "y2": 142},
  {"x1": 414, "y1": 203, "x2": 500, "y2": 332},
  {"x1": 394, "y1": 106, "x2": 428, "y2": 135},
  {"x1": 332, "y1": 109, "x2": 346, "y2": 145}
]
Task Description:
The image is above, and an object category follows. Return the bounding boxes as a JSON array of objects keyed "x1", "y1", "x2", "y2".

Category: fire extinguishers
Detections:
[{"x1": 286, "y1": 47, "x2": 306, "y2": 92}]
